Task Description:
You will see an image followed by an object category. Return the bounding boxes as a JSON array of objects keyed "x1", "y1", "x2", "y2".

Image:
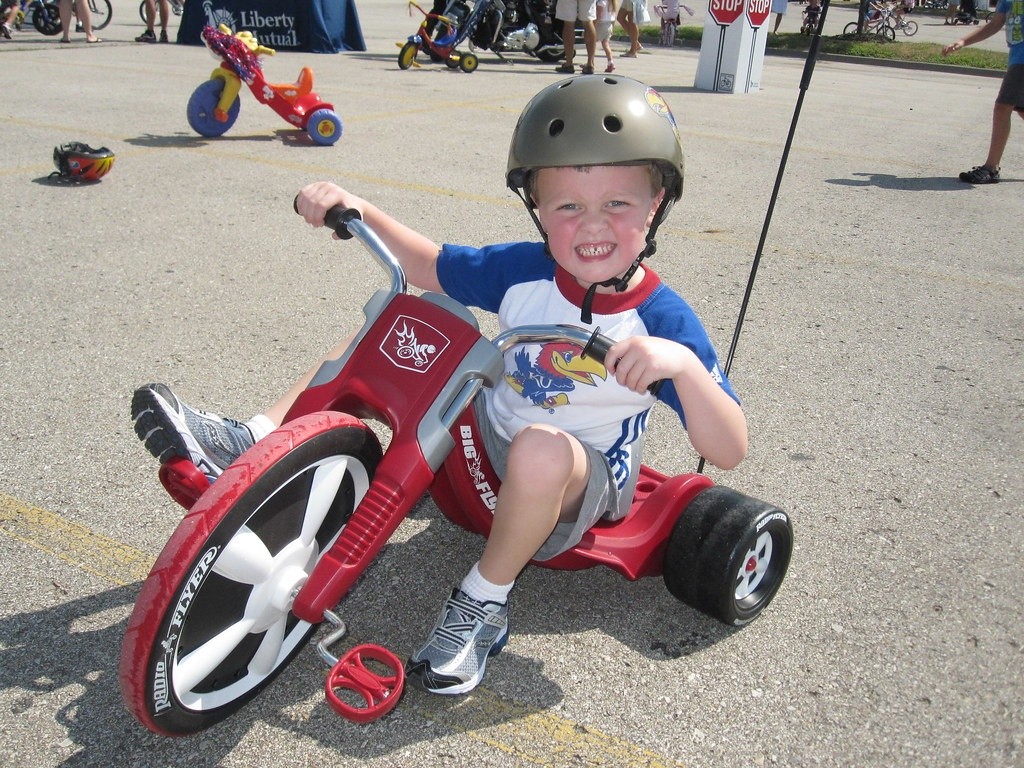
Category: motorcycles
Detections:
[{"x1": 466, "y1": 0, "x2": 576, "y2": 65}]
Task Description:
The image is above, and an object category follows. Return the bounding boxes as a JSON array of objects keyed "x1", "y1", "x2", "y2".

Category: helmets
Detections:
[
  {"x1": 52, "y1": 140, "x2": 116, "y2": 181},
  {"x1": 505, "y1": 72, "x2": 685, "y2": 212}
]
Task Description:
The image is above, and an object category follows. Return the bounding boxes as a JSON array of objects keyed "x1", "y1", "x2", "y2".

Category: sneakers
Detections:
[
  {"x1": 960, "y1": 163, "x2": 1003, "y2": 183},
  {"x1": 129, "y1": 380, "x2": 256, "y2": 492},
  {"x1": 159, "y1": 33, "x2": 169, "y2": 43},
  {"x1": 135, "y1": 30, "x2": 156, "y2": 42},
  {"x1": 404, "y1": 583, "x2": 511, "y2": 693}
]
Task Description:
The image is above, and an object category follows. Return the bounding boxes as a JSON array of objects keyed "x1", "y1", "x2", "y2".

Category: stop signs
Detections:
[
  {"x1": 745, "y1": 0, "x2": 772, "y2": 28},
  {"x1": 709, "y1": 0, "x2": 745, "y2": 26}
]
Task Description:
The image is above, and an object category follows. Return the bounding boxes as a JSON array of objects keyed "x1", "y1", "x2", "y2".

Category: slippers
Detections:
[
  {"x1": 61, "y1": 38, "x2": 72, "y2": 43},
  {"x1": 86, "y1": 37, "x2": 102, "y2": 43}
]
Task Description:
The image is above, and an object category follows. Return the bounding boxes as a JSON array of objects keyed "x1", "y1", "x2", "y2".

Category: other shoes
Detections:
[
  {"x1": 619, "y1": 52, "x2": 636, "y2": 57},
  {"x1": 605, "y1": 63, "x2": 616, "y2": 72},
  {"x1": 893, "y1": 25, "x2": 900, "y2": 30},
  {"x1": 582, "y1": 65, "x2": 594, "y2": 74},
  {"x1": 636, "y1": 46, "x2": 643, "y2": 50},
  {"x1": 944, "y1": 22, "x2": 951, "y2": 25},
  {"x1": 2, "y1": 24, "x2": 12, "y2": 40},
  {"x1": 555, "y1": 63, "x2": 575, "y2": 73}
]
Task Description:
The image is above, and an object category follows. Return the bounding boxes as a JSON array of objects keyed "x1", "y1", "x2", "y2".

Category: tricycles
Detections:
[
  {"x1": 0, "y1": 0, "x2": 64, "y2": 36},
  {"x1": 187, "y1": 23, "x2": 342, "y2": 147},
  {"x1": 395, "y1": 0, "x2": 490, "y2": 73},
  {"x1": 800, "y1": 10, "x2": 822, "y2": 35},
  {"x1": 116, "y1": 193, "x2": 798, "y2": 738}
]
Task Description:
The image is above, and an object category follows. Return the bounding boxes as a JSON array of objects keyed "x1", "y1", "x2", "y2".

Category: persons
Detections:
[
  {"x1": 58, "y1": 0, "x2": 103, "y2": 43},
  {"x1": 135, "y1": 0, "x2": 169, "y2": 42},
  {"x1": 771, "y1": 0, "x2": 979, "y2": 38},
  {"x1": 131, "y1": 74, "x2": 749, "y2": 694},
  {"x1": 942, "y1": 0, "x2": 1024, "y2": 184},
  {"x1": 0, "y1": 0, "x2": 20, "y2": 40},
  {"x1": 554, "y1": 0, "x2": 681, "y2": 74}
]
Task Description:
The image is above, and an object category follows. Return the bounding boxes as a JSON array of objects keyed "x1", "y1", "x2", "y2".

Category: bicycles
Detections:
[
  {"x1": 657, "y1": 6, "x2": 676, "y2": 46},
  {"x1": 71, "y1": 0, "x2": 112, "y2": 30},
  {"x1": 985, "y1": 11, "x2": 996, "y2": 23},
  {"x1": 844, "y1": 6, "x2": 918, "y2": 41},
  {"x1": 139, "y1": 0, "x2": 185, "y2": 27}
]
